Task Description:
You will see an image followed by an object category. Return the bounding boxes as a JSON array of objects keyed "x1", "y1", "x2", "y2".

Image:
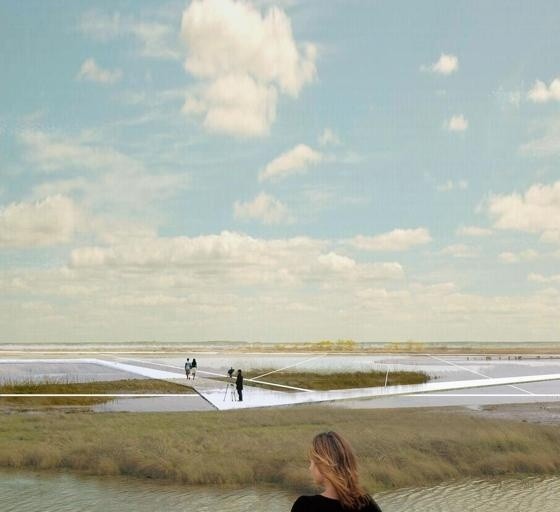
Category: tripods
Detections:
[{"x1": 223, "y1": 375, "x2": 236, "y2": 402}]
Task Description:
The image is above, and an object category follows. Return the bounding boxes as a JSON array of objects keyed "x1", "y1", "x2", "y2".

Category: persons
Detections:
[
  {"x1": 288, "y1": 428, "x2": 383, "y2": 511},
  {"x1": 185, "y1": 358, "x2": 191, "y2": 380},
  {"x1": 235, "y1": 369, "x2": 243, "y2": 401},
  {"x1": 191, "y1": 358, "x2": 197, "y2": 380}
]
